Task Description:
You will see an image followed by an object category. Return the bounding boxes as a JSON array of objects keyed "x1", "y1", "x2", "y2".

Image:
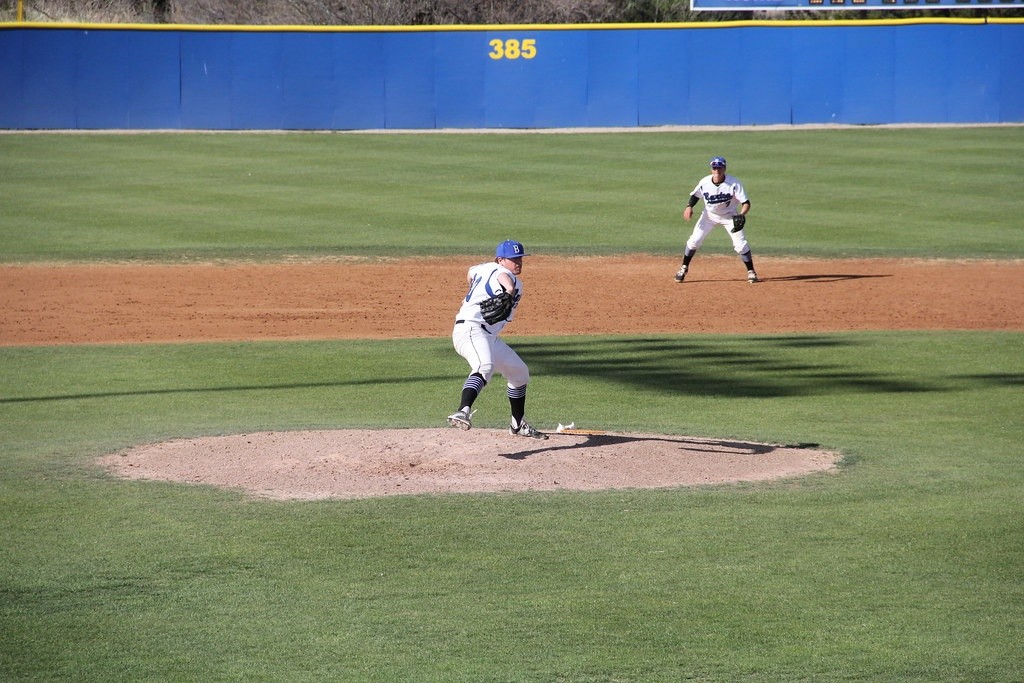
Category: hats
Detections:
[
  {"x1": 495, "y1": 239, "x2": 531, "y2": 259},
  {"x1": 709, "y1": 156, "x2": 726, "y2": 167}
]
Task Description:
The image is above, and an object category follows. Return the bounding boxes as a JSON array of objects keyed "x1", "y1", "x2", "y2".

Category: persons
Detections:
[
  {"x1": 446, "y1": 238, "x2": 550, "y2": 440},
  {"x1": 674, "y1": 155, "x2": 758, "y2": 284}
]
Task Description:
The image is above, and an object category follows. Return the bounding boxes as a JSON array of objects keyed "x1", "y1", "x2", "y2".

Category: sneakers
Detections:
[
  {"x1": 674, "y1": 265, "x2": 688, "y2": 283},
  {"x1": 509, "y1": 416, "x2": 550, "y2": 440},
  {"x1": 446, "y1": 406, "x2": 480, "y2": 431},
  {"x1": 747, "y1": 270, "x2": 757, "y2": 284}
]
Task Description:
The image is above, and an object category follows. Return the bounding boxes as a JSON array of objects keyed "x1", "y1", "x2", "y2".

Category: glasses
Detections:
[{"x1": 710, "y1": 160, "x2": 726, "y2": 166}]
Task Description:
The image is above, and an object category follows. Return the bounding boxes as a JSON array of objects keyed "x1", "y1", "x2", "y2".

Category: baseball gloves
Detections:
[
  {"x1": 729, "y1": 214, "x2": 746, "y2": 233},
  {"x1": 478, "y1": 292, "x2": 514, "y2": 326}
]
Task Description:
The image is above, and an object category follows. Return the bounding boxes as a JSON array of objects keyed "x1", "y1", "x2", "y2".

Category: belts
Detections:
[{"x1": 456, "y1": 320, "x2": 492, "y2": 334}]
